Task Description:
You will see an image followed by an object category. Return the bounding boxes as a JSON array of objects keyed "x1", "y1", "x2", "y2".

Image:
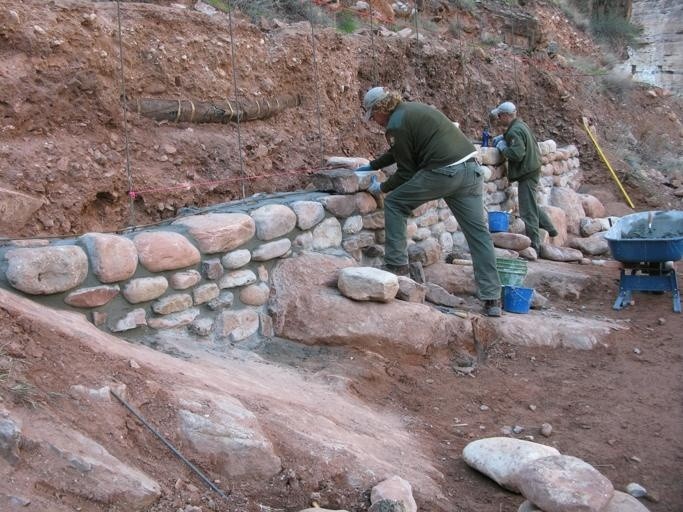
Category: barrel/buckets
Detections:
[
  {"x1": 488, "y1": 211, "x2": 511, "y2": 232},
  {"x1": 501, "y1": 285, "x2": 533, "y2": 314},
  {"x1": 497, "y1": 256, "x2": 527, "y2": 285}
]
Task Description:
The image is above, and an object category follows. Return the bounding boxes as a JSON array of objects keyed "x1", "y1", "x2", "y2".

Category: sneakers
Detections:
[
  {"x1": 478, "y1": 300, "x2": 501, "y2": 317},
  {"x1": 373, "y1": 264, "x2": 410, "y2": 278},
  {"x1": 548, "y1": 229, "x2": 558, "y2": 237}
]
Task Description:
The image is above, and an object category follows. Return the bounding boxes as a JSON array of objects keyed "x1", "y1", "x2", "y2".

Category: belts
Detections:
[{"x1": 466, "y1": 157, "x2": 478, "y2": 163}]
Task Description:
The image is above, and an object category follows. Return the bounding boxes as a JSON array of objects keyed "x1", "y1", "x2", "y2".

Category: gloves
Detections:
[
  {"x1": 366, "y1": 175, "x2": 382, "y2": 197},
  {"x1": 492, "y1": 134, "x2": 508, "y2": 152},
  {"x1": 355, "y1": 163, "x2": 374, "y2": 171}
]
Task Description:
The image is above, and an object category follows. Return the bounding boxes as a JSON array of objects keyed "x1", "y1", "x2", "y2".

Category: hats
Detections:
[
  {"x1": 490, "y1": 102, "x2": 516, "y2": 115},
  {"x1": 363, "y1": 86, "x2": 390, "y2": 123}
]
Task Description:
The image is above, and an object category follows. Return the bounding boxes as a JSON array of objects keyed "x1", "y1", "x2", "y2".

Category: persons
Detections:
[
  {"x1": 345, "y1": 85, "x2": 502, "y2": 317},
  {"x1": 489, "y1": 99, "x2": 560, "y2": 262}
]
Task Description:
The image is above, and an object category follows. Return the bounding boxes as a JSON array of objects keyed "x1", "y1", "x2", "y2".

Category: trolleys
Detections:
[{"x1": 604, "y1": 209, "x2": 683, "y2": 313}]
